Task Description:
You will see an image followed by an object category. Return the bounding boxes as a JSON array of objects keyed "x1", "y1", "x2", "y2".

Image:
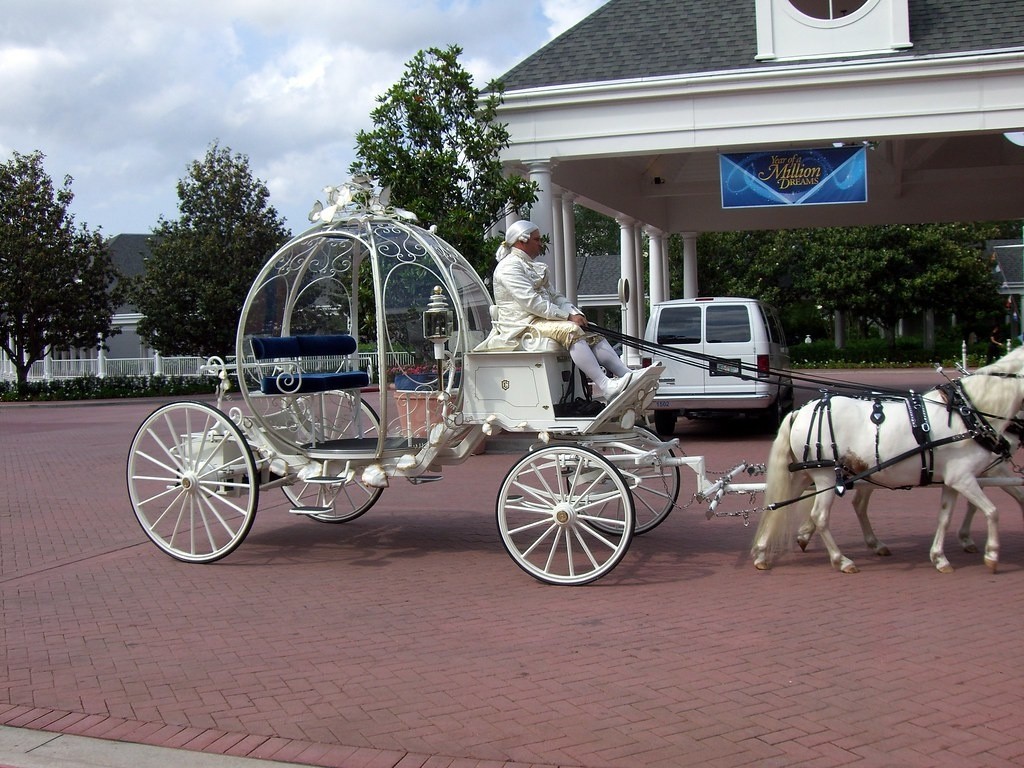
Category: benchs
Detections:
[
  {"x1": 395, "y1": 373, "x2": 459, "y2": 447},
  {"x1": 489, "y1": 305, "x2": 562, "y2": 351},
  {"x1": 246, "y1": 336, "x2": 369, "y2": 397}
]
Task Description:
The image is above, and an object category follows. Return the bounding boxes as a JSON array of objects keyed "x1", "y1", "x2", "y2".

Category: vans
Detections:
[{"x1": 641, "y1": 297, "x2": 794, "y2": 437}]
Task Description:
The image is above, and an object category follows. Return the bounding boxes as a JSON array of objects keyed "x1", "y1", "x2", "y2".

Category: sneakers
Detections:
[
  {"x1": 601, "y1": 372, "x2": 633, "y2": 402},
  {"x1": 628, "y1": 361, "x2": 662, "y2": 387}
]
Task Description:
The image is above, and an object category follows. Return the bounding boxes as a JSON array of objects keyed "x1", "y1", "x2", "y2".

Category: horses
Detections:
[{"x1": 750, "y1": 343, "x2": 1024, "y2": 574}]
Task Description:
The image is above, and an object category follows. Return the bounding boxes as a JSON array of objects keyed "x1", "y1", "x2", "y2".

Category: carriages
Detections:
[{"x1": 126, "y1": 174, "x2": 1024, "y2": 586}]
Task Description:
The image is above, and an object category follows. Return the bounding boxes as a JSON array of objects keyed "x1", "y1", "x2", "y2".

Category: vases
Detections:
[{"x1": 393, "y1": 392, "x2": 463, "y2": 438}]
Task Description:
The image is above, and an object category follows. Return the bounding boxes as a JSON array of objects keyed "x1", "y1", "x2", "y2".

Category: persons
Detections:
[
  {"x1": 474, "y1": 220, "x2": 662, "y2": 402},
  {"x1": 986, "y1": 325, "x2": 1003, "y2": 366}
]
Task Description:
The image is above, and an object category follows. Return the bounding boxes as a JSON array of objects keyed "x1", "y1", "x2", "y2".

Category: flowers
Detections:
[{"x1": 386, "y1": 366, "x2": 461, "y2": 374}]
